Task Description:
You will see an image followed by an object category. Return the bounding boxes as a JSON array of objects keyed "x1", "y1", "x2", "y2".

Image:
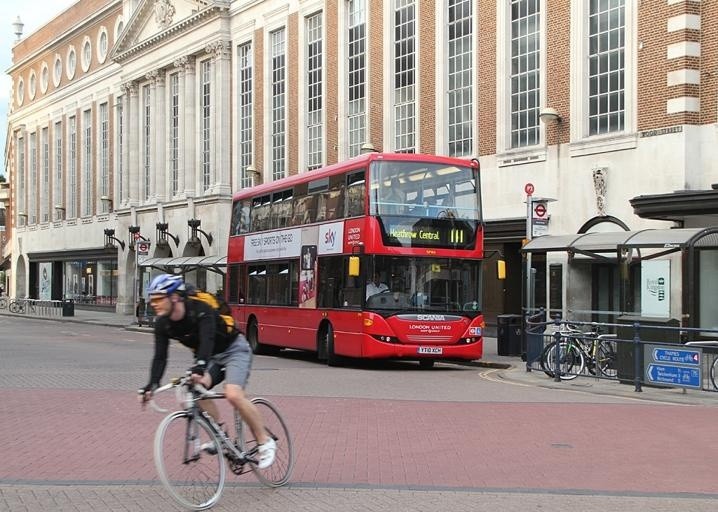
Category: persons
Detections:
[
  {"x1": 383, "y1": 177, "x2": 405, "y2": 214},
  {"x1": 302, "y1": 247, "x2": 313, "y2": 270},
  {"x1": 365, "y1": 269, "x2": 390, "y2": 303},
  {"x1": 138, "y1": 274, "x2": 277, "y2": 471}
]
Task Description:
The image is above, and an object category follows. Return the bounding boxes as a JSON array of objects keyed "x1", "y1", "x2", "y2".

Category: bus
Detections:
[{"x1": 224, "y1": 151, "x2": 506, "y2": 367}]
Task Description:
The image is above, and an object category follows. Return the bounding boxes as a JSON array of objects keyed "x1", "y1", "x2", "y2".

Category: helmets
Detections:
[{"x1": 148, "y1": 274, "x2": 184, "y2": 299}]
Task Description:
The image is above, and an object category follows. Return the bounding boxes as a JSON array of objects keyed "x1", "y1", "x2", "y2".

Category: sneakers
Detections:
[
  {"x1": 258, "y1": 438, "x2": 276, "y2": 468},
  {"x1": 201, "y1": 443, "x2": 215, "y2": 452}
]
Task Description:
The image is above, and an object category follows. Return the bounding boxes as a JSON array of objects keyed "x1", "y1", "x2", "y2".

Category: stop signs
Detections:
[
  {"x1": 532, "y1": 202, "x2": 548, "y2": 219},
  {"x1": 139, "y1": 244, "x2": 148, "y2": 253}
]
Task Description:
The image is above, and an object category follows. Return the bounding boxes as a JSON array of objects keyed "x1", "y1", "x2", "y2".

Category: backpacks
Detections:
[{"x1": 186, "y1": 288, "x2": 235, "y2": 333}]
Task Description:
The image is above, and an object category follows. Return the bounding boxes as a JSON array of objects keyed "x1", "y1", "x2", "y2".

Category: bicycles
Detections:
[
  {"x1": 540, "y1": 320, "x2": 619, "y2": 380},
  {"x1": 0, "y1": 298, "x2": 8, "y2": 310},
  {"x1": 9, "y1": 297, "x2": 36, "y2": 314},
  {"x1": 709, "y1": 355, "x2": 718, "y2": 390},
  {"x1": 142, "y1": 370, "x2": 295, "y2": 511}
]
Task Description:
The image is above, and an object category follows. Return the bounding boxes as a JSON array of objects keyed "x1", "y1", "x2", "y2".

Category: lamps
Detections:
[
  {"x1": 18, "y1": 195, "x2": 114, "y2": 217},
  {"x1": 361, "y1": 143, "x2": 380, "y2": 153},
  {"x1": 537, "y1": 108, "x2": 562, "y2": 123},
  {"x1": 246, "y1": 165, "x2": 261, "y2": 178},
  {"x1": 102, "y1": 218, "x2": 213, "y2": 252}
]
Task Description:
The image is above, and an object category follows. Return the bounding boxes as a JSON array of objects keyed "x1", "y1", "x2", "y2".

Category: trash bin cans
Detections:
[
  {"x1": 495, "y1": 314, "x2": 523, "y2": 357},
  {"x1": 62, "y1": 298, "x2": 75, "y2": 317}
]
